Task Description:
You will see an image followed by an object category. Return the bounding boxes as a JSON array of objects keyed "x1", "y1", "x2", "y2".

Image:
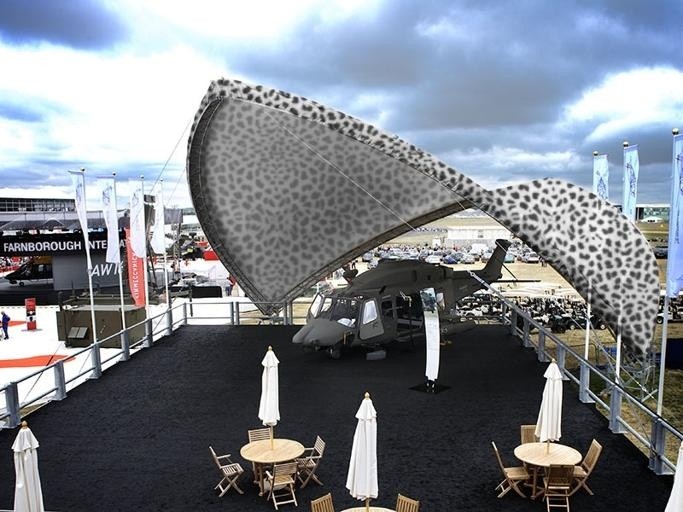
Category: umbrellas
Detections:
[
  {"x1": 535, "y1": 358, "x2": 563, "y2": 453},
  {"x1": 10, "y1": 420, "x2": 44, "y2": 512},
  {"x1": 258, "y1": 346, "x2": 280, "y2": 449},
  {"x1": 345, "y1": 392, "x2": 379, "y2": 512}
]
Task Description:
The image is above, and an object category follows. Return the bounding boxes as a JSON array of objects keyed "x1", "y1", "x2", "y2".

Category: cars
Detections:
[{"x1": 361, "y1": 244, "x2": 538, "y2": 264}]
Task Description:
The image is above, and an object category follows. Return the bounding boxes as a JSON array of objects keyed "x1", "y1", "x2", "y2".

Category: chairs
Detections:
[
  {"x1": 247, "y1": 426, "x2": 275, "y2": 485},
  {"x1": 395, "y1": 493, "x2": 420, "y2": 512},
  {"x1": 540, "y1": 463, "x2": 574, "y2": 512},
  {"x1": 293, "y1": 435, "x2": 326, "y2": 490},
  {"x1": 519, "y1": 423, "x2": 540, "y2": 470},
  {"x1": 310, "y1": 493, "x2": 334, "y2": 512},
  {"x1": 264, "y1": 461, "x2": 299, "y2": 512},
  {"x1": 208, "y1": 445, "x2": 246, "y2": 498},
  {"x1": 490, "y1": 441, "x2": 529, "y2": 501},
  {"x1": 566, "y1": 438, "x2": 602, "y2": 498}
]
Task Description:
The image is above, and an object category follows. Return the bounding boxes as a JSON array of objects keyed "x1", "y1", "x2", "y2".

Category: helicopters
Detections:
[
  {"x1": 2, "y1": 254, "x2": 52, "y2": 287},
  {"x1": 291, "y1": 236, "x2": 512, "y2": 362}
]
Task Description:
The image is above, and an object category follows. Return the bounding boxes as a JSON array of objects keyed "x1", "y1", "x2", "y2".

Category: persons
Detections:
[
  {"x1": 0, "y1": 255, "x2": 32, "y2": 275},
  {"x1": 1, "y1": 312, "x2": 11, "y2": 339}
]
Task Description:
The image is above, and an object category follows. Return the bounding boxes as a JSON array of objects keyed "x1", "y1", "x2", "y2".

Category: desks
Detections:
[
  {"x1": 239, "y1": 439, "x2": 305, "y2": 498},
  {"x1": 512, "y1": 441, "x2": 582, "y2": 502},
  {"x1": 338, "y1": 506, "x2": 396, "y2": 512}
]
